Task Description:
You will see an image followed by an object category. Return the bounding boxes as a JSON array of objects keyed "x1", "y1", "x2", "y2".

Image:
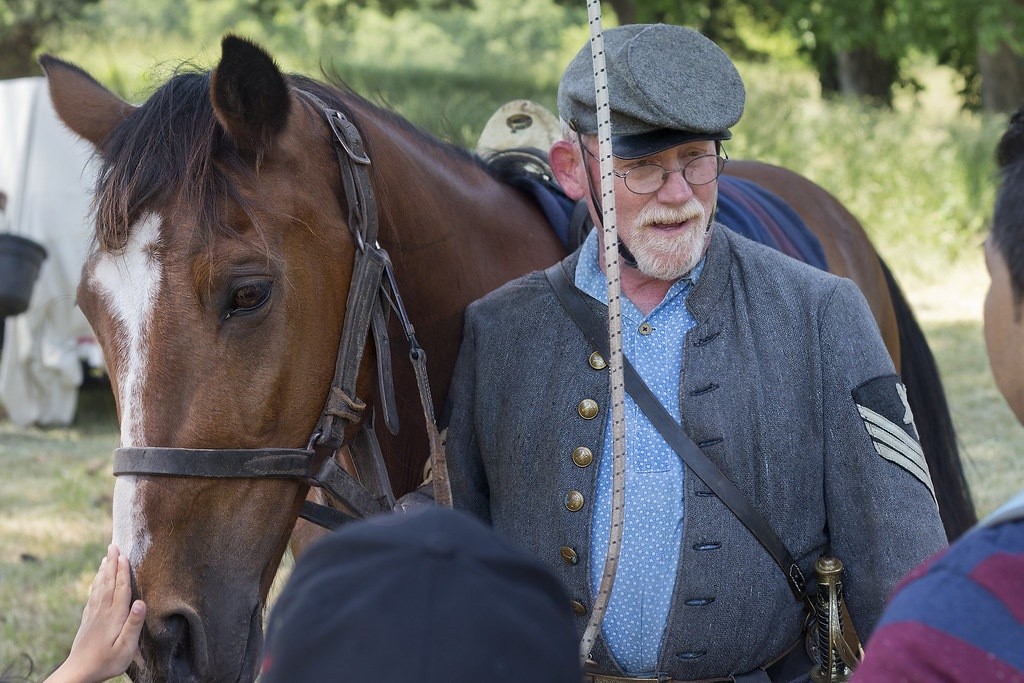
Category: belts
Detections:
[{"x1": 585, "y1": 628, "x2": 814, "y2": 683}]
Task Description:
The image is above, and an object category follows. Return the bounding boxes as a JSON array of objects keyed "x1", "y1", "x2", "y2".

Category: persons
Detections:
[
  {"x1": 252, "y1": 502, "x2": 583, "y2": 682},
  {"x1": 846, "y1": 104, "x2": 1024, "y2": 683},
  {"x1": 390, "y1": 23, "x2": 948, "y2": 683},
  {"x1": 40, "y1": 543, "x2": 146, "y2": 683}
]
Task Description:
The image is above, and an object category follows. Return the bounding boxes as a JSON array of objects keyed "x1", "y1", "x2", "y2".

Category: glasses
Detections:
[{"x1": 572, "y1": 139, "x2": 729, "y2": 195}]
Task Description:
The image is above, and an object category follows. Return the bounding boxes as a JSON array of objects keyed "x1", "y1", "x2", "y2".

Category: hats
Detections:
[
  {"x1": 264, "y1": 504, "x2": 580, "y2": 682},
  {"x1": 558, "y1": 23, "x2": 747, "y2": 160}
]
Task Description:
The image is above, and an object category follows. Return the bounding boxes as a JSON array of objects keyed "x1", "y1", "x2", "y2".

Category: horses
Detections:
[{"x1": 38, "y1": 33, "x2": 980, "y2": 683}]
